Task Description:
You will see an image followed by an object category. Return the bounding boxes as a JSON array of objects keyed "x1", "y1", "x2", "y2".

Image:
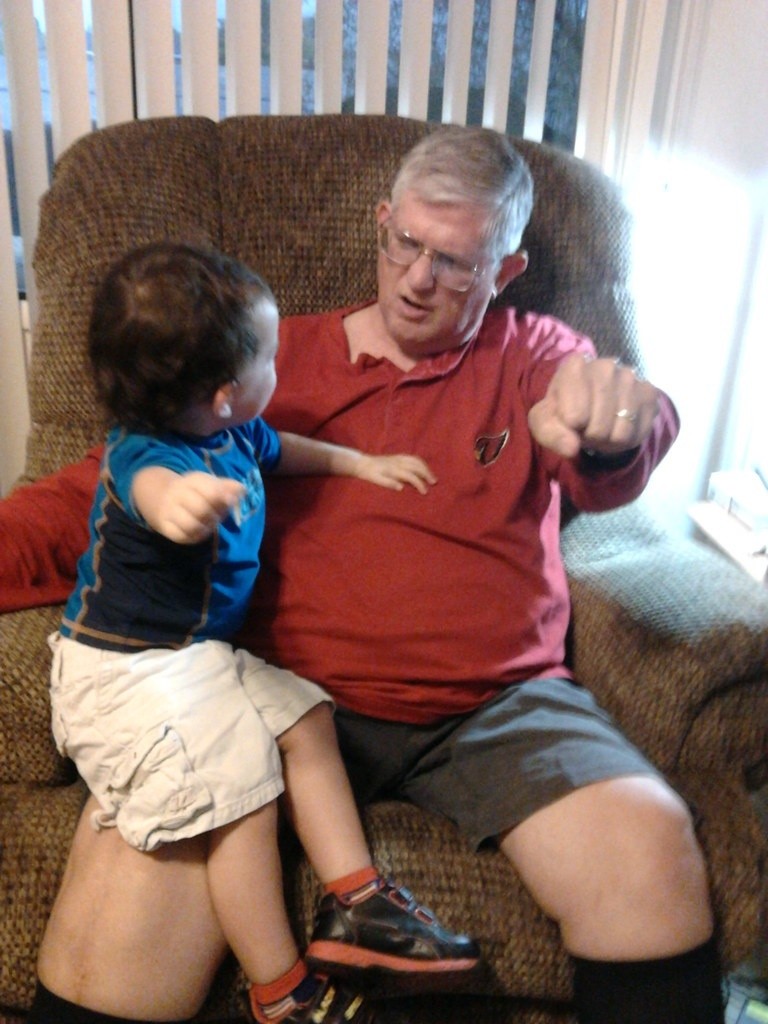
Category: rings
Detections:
[{"x1": 617, "y1": 409, "x2": 638, "y2": 421}]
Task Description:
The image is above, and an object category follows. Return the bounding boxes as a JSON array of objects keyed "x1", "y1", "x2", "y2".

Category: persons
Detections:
[
  {"x1": 46, "y1": 241, "x2": 481, "y2": 1024},
  {"x1": 0, "y1": 123, "x2": 725, "y2": 1023}
]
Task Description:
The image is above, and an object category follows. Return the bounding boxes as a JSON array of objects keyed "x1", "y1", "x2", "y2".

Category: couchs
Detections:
[{"x1": 0, "y1": 112, "x2": 768, "y2": 1024}]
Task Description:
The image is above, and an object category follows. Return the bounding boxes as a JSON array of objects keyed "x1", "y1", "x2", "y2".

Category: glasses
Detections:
[{"x1": 380, "y1": 222, "x2": 506, "y2": 293}]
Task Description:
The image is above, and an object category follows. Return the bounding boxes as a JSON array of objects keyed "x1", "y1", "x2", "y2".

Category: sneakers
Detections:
[
  {"x1": 234, "y1": 977, "x2": 400, "y2": 1024},
  {"x1": 308, "y1": 879, "x2": 480, "y2": 974}
]
